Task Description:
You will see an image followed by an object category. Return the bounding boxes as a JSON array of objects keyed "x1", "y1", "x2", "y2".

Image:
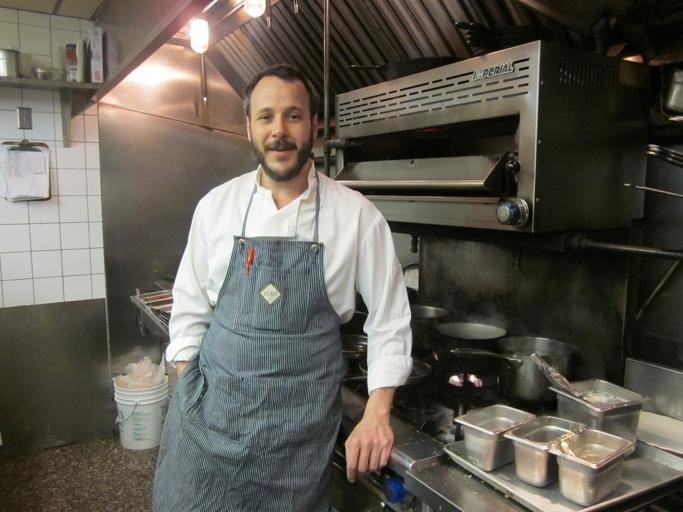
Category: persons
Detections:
[{"x1": 148, "y1": 63, "x2": 417, "y2": 512}]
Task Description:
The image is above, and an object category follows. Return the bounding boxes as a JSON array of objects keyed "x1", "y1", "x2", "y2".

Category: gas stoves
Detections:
[{"x1": 341, "y1": 342, "x2": 589, "y2": 480}]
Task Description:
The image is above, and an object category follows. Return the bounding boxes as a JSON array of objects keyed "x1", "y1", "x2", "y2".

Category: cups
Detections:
[{"x1": 34, "y1": 70, "x2": 48, "y2": 81}]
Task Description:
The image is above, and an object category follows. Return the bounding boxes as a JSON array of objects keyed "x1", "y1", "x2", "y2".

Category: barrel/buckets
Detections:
[{"x1": 110, "y1": 376, "x2": 171, "y2": 452}]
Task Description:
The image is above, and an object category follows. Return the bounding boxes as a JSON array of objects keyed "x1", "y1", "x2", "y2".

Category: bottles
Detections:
[{"x1": 66, "y1": 44, "x2": 79, "y2": 83}]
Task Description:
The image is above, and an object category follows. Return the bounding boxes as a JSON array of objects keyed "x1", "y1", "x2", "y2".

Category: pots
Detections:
[
  {"x1": 343, "y1": 54, "x2": 464, "y2": 80},
  {"x1": 448, "y1": 324, "x2": 572, "y2": 404},
  {"x1": 454, "y1": 16, "x2": 553, "y2": 57},
  {"x1": 341, "y1": 354, "x2": 434, "y2": 392},
  {"x1": 0, "y1": 48, "x2": 20, "y2": 77},
  {"x1": 339, "y1": 331, "x2": 368, "y2": 361},
  {"x1": 409, "y1": 303, "x2": 452, "y2": 333},
  {"x1": 409, "y1": 317, "x2": 508, "y2": 362}
]
